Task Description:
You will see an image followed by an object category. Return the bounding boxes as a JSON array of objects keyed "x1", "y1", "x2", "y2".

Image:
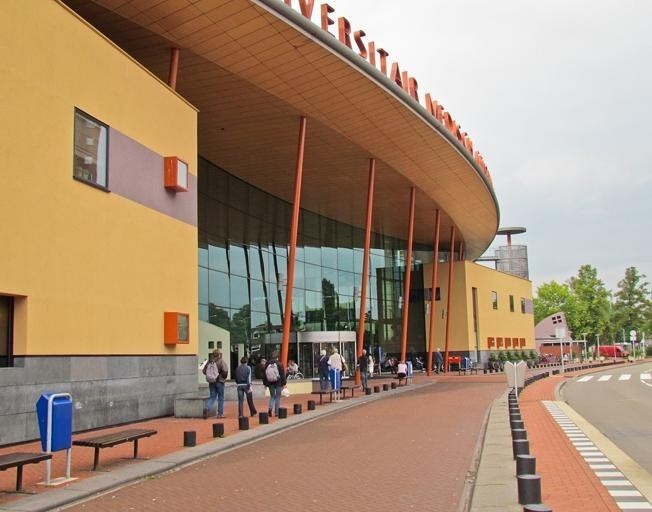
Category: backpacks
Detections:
[
  {"x1": 265, "y1": 361, "x2": 280, "y2": 382},
  {"x1": 206, "y1": 358, "x2": 221, "y2": 383}
]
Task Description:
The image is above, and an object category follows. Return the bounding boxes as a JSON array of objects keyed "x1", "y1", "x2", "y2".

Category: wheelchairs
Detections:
[{"x1": 288, "y1": 367, "x2": 305, "y2": 380}]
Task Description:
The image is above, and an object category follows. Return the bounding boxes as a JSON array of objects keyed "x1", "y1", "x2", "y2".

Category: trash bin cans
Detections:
[
  {"x1": 330, "y1": 368, "x2": 341, "y2": 401},
  {"x1": 36, "y1": 393, "x2": 78, "y2": 486}
]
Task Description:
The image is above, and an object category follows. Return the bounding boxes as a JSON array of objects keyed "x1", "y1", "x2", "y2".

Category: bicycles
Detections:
[{"x1": 492, "y1": 360, "x2": 504, "y2": 370}]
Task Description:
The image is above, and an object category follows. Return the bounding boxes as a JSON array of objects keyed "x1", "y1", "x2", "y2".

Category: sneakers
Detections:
[
  {"x1": 204, "y1": 407, "x2": 228, "y2": 419},
  {"x1": 269, "y1": 410, "x2": 279, "y2": 417},
  {"x1": 239, "y1": 412, "x2": 259, "y2": 418}
]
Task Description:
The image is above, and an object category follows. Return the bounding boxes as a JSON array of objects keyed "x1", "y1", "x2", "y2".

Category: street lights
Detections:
[
  {"x1": 568, "y1": 330, "x2": 573, "y2": 360},
  {"x1": 595, "y1": 334, "x2": 601, "y2": 357},
  {"x1": 581, "y1": 333, "x2": 588, "y2": 361}
]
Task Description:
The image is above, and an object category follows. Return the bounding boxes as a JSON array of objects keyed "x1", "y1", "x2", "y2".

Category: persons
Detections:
[
  {"x1": 413, "y1": 356, "x2": 424, "y2": 372},
  {"x1": 233, "y1": 356, "x2": 258, "y2": 419},
  {"x1": 262, "y1": 350, "x2": 287, "y2": 417},
  {"x1": 434, "y1": 346, "x2": 443, "y2": 375},
  {"x1": 203, "y1": 348, "x2": 228, "y2": 419},
  {"x1": 316, "y1": 347, "x2": 408, "y2": 394},
  {"x1": 260, "y1": 355, "x2": 299, "y2": 380}
]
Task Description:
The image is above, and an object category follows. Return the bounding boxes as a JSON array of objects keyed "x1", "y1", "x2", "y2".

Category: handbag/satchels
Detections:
[{"x1": 342, "y1": 362, "x2": 346, "y2": 371}]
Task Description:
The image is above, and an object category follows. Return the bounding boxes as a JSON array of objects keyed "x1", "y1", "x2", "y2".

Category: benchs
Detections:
[
  {"x1": 0, "y1": 450, "x2": 54, "y2": 491},
  {"x1": 309, "y1": 384, "x2": 362, "y2": 404},
  {"x1": 72, "y1": 427, "x2": 157, "y2": 472},
  {"x1": 393, "y1": 375, "x2": 415, "y2": 385}
]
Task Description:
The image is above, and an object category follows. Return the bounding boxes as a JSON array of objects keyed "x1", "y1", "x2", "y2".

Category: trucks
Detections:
[{"x1": 593, "y1": 344, "x2": 629, "y2": 356}]
